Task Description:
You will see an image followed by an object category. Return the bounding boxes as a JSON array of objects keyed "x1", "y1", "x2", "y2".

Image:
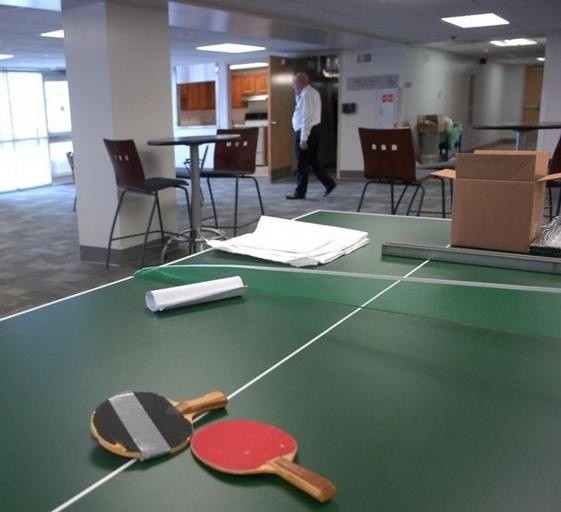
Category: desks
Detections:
[
  {"x1": 147, "y1": 134, "x2": 241, "y2": 256},
  {"x1": 472, "y1": 125, "x2": 561, "y2": 150},
  {"x1": 0, "y1": 209, "x2": 561, "y2": 512}
]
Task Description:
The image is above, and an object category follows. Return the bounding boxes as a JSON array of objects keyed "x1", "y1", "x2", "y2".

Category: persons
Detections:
[{"x1": 284, "y1": 71, "x2": 338, "y2": 200}]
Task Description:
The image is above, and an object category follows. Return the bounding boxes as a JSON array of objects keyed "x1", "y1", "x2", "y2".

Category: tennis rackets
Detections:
[
  {"x1": 190, "y1": 421, "x2": 337, "y2": 503},
  {"x1": 90, "y1": 390, "x2": 227, "y2": 460}
]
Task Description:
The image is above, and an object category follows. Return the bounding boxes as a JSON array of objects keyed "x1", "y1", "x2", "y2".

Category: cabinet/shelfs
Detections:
[
  {"x1": 231, "y1": 71, "x2": 269, "y2": 108},
  {"x1": 178, "y1": 82, "x2": 215, "y2": 110}
]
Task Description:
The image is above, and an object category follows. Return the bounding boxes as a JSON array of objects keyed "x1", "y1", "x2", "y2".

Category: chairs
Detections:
[
  {"x1": 102, "y1": 139, "x2": 193, "y2": 271},
  {"x1": 201, "y1": 128, "x2": 264, "y2": 238},
  {"x1": 176, "y1": 146, "x2": 208, "y2": 206},
  {"x1": 543, "y1": 137, "x2": 561, "y2": 222},
  {"x1": 358, "y1": 126, "x2": 424, "y2": 216}
]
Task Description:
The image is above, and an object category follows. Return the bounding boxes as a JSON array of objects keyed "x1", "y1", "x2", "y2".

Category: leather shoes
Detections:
[
  {"x1": 286, "y1": 192, "x2": 306, "y2": 199},
  {"x1": 324, "y1": 183, "x2": 337, "y2": 197}
]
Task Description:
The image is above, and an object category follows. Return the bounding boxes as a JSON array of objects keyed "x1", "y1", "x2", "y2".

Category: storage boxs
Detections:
[{"x1": 431, "y1": 149, "x2": 561, "y2": 253}]
